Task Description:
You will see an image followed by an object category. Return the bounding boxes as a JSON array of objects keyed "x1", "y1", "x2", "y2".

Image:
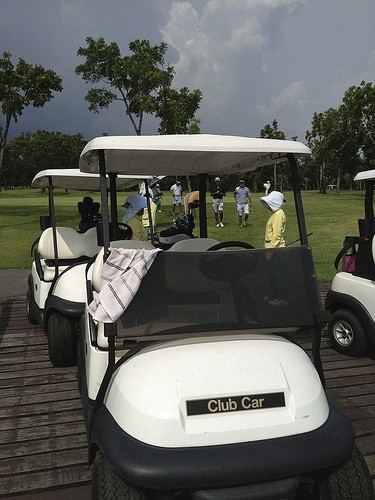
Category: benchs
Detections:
[
  {"x1": 38, "y1": 227, "x2": 98, "y2": 282},
  {"x1": 93, "y1": 239, "x2": 227, "y2": 349}
]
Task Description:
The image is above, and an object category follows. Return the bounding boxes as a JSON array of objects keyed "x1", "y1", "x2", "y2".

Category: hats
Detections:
[
  {"x1": 260, "y1": 191, "x2": 284, "y2": 213},
  {"x1": 156, "y1": 184, "x2": 160, "y2": 187},
  {"x1": 176, "y1": 180, "x2": 181, "y2": 183},
  {"x1": 215, "y1": 177, "x2": 220, "y2": 182},
  {"x1": 121, "y1": 192, "x2": 147, "y2": 226},
  {"x1": 239, "y1": 180, "x2": 244, "y2": 183}
]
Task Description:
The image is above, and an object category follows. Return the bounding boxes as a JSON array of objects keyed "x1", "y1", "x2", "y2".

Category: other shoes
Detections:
[
  {"x1": 243, "y1": 222, "x2": 246, "y2": 226},
  {"x1": 220, "y1": 223, "x2": 225, "y2": 227},
  {"x1": 180, "y1": 211, "x2": 184, "y2": 215},
  {"x1": 216, "y1": 224, "x2": 220, "y2": 227},
  {"x1": 240, "y1": 224, "x2": 243, "y2": 227},
  {"x1": 173, "y1": 211, "x2": 175, "y2": 215},
  {"x1": 158, "y1": 210, "x2": 161, "y2": 212}
]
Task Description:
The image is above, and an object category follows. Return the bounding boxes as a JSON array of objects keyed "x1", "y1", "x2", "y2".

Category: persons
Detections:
[
  {"x1": 210, "y1": 176, "x2": 225, "y2": 227},
  {"x1": 233, "y1": 180, "x2": 253, "y2": 227},
  {"x1": 184, "y1": 191, "x2": 200, "y2": 216},
  {"x1": 260, "y1": 190, "x2": 287, "y2": 260},
  {"x1": 140, "y1": 182, "x2": 164, "y2": 236},
  {"x1": 122, "y1": 194, "x2": 152, "y2": 242},
  {"x1": 169, "y1": 180, "x2": 184, "y2": 215},
  {"x1": 263, "y1": 180, "x2": 271, "y2": 196}
]
